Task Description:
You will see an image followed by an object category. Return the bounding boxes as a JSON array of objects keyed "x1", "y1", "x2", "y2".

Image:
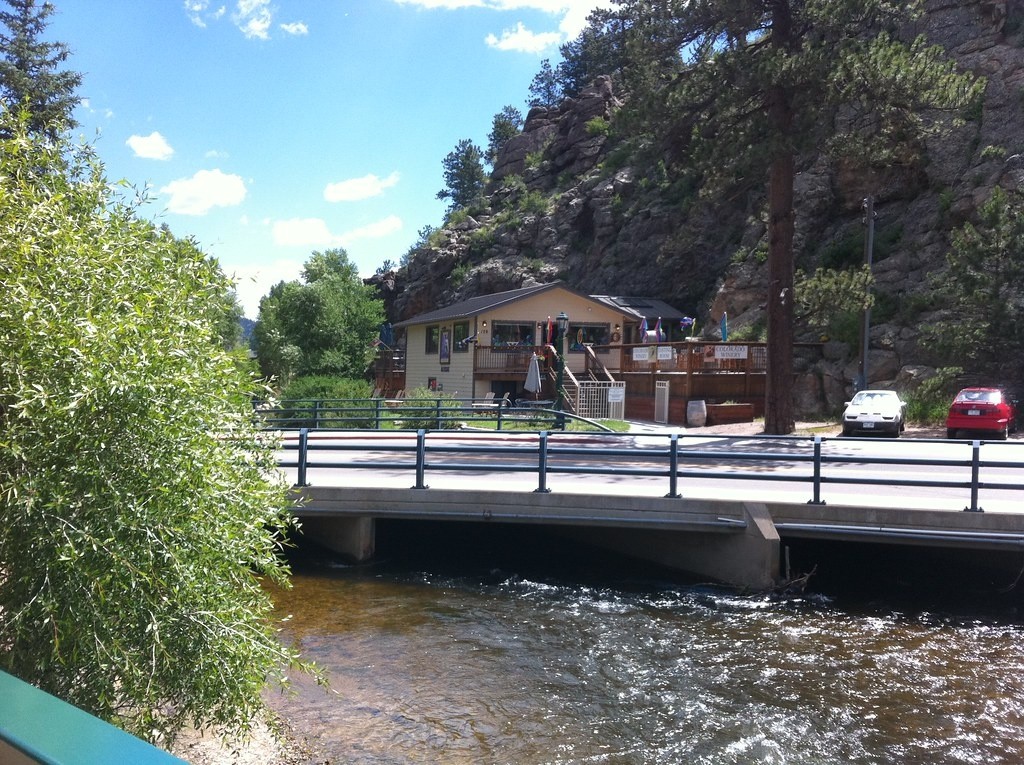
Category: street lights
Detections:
[{"x1": 552, "y1": 311, "x2": 570, "y2": 428}]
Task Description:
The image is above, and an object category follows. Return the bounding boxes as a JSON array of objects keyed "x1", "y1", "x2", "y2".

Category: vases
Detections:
[{"x1": 686, "y1": 400, "x2": 707, "y2": 427}]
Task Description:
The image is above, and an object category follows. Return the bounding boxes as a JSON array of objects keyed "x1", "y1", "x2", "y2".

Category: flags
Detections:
[
  {"x1": 639, "y1": 318, "x2": 648, "y2": 344},
  {"x1": 679, "y1": 317, "x2": 696, "y2": 331},
  {"x1": 655, "y1": 319, "x2": 661, "y2": 342},
  {"x1": 546, "y1": 318, "x2": 553, "y2": 344},
  {"x1": 367, "y1": 337, "x2": 382, "y2": 348}
]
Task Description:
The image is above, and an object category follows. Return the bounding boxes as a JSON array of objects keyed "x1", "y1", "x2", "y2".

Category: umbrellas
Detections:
[{"x1": 524, "y1": 352, "x2": 541, "y2": 400}]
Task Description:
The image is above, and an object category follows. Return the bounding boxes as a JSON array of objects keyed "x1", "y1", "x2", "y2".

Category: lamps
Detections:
[
  {"x1": 614, "y1": 323, "x2": 621, "y2": 331},
  {"x1": 538, "y1": 322, "x2": 542, "y2": 329},
  {"x1": 482, "y1": 320, "x2": 487, "y2": 327}
]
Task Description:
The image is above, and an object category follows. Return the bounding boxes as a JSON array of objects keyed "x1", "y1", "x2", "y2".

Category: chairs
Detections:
[{"x1": 471, "y1": 391, "x2": 510, "y2": 418}]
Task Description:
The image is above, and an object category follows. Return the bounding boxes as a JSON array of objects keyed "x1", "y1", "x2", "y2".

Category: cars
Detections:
[
  {"x1": 842, "y1": 390, "x2": 907, "y2": 439},
  {"x1": 947, "y1": 387, "x2": 1020, "y2": 440}
]
